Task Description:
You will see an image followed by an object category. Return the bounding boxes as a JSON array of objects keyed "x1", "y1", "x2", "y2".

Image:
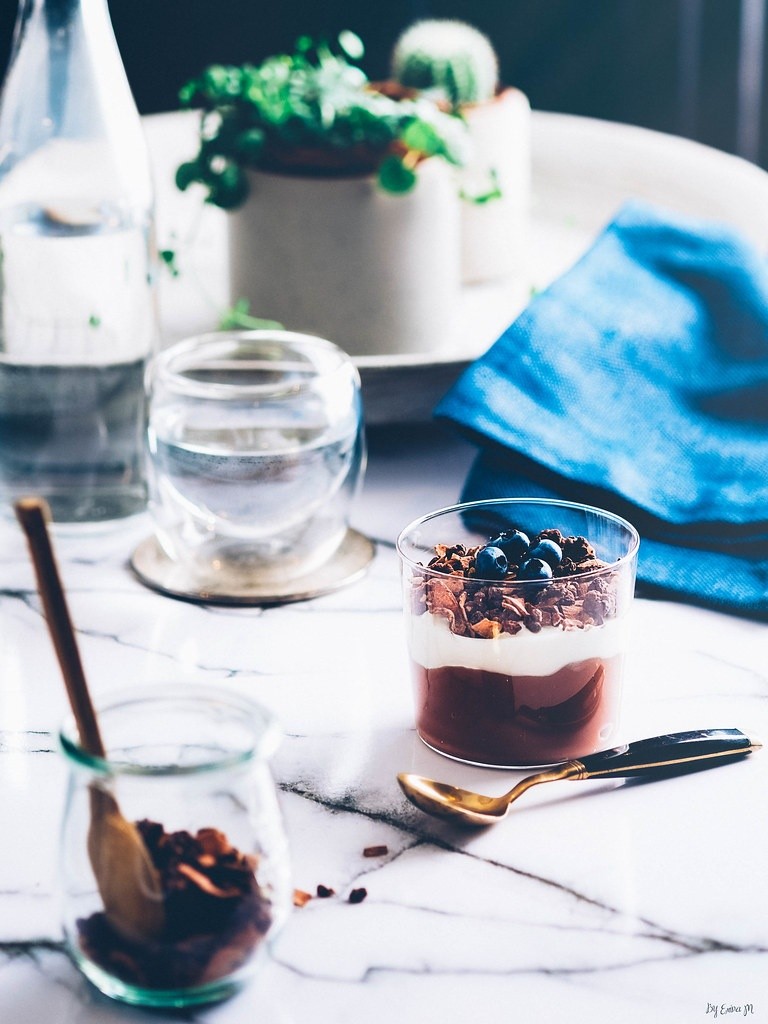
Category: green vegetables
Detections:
[{"x1": 166, "y1": 31, "x2": 506, "y2": 335}]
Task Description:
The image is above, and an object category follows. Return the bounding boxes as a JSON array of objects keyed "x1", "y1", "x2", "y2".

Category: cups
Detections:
[
  {"x1": 58, "y1": 688, "x2": 295, "y2": 1009},
  {"x1": 396, "y1": 497, "x2": 642, "y2": 774}
]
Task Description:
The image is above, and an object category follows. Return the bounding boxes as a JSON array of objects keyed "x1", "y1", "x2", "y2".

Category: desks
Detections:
[{"x1": 0, "y1": 102, "x2": 768, "y2": 1023}]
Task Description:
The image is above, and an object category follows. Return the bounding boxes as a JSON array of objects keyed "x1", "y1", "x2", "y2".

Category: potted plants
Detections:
[{"x1": 174, "y1": 18, "x2": 529, "y2": 358}]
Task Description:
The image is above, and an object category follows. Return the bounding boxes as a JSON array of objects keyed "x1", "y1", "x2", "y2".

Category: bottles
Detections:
[{"x1": 0, "y1": 0, "x2": 160, "y2": 526}]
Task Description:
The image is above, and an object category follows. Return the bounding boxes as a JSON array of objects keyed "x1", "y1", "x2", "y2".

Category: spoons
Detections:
[
  {"x1": 16, "y1": 496, "x2": 167, "y2": 943},
  {"x1": 397, "y1": 727, "x2": 764, "y2": 827}
]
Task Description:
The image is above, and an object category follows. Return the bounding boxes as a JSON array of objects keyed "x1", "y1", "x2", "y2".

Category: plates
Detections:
[{"x1": 128, "y1": 526, "x2": 377, "y2": 608}]
